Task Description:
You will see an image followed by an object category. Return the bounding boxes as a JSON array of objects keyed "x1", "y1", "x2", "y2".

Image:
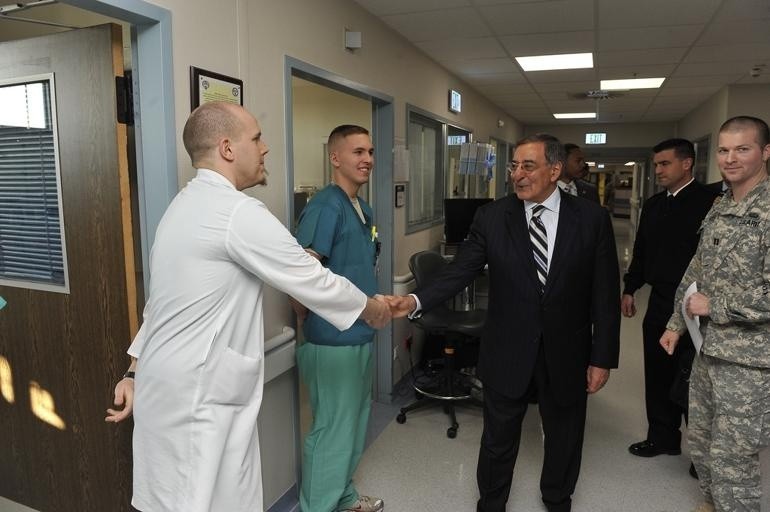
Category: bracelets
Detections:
[{"x1": 122, "y1": 371, "x2": 136, "y2": 379}]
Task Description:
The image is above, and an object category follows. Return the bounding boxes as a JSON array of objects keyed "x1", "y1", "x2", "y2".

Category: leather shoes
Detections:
[{"x1": 628, "y1": 439, "x2": 683, "y2": 457}]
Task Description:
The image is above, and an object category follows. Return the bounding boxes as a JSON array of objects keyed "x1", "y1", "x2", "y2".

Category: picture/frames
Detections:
[{"x1": 189, "y1": 63, "x2": 242, "y2": 116}]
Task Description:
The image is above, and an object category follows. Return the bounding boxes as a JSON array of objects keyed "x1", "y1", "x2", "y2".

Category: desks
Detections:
[{"x1": 438, "y1": 242, "x2": 492, "y2": 390}]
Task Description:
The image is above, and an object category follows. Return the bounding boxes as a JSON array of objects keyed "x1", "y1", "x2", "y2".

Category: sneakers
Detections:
[{"x1": 342, "y1": 494, "x2": 384, "y2": 512}]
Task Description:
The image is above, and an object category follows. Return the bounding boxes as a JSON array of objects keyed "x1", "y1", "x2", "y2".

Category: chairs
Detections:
[{"x1": 395, "y1": 245, "x2": 496, "y2": 440}]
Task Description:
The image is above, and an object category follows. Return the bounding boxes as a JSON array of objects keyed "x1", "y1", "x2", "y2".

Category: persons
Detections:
[
  {"x1": 659, "y1": 116, "x2": 770, "y2": 511},
  {"x1": 288, "y1": 125, "x2": 384, "y2": 511},
  {"x1": 559, "y1": 143, "x2": 600, "y2": 205},
  {"x1": 376, "y1": 131, "x2": 622, "y2": 512},
  {"x1": 104, "y1": 100, "x2": 391, "y2": 512},
  {"x1": 620, "y1": 136, "x2": 720, "y2": 481}
]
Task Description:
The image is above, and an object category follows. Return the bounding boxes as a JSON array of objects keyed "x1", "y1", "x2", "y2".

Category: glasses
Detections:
[{"x1": 506, "y1": 161, "x2": 540, "y2": 173}]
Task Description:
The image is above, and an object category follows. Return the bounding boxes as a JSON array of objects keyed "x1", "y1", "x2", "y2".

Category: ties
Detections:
[{"x1": 528, "y1": 204, "x2": 549, "y2": 293}]
